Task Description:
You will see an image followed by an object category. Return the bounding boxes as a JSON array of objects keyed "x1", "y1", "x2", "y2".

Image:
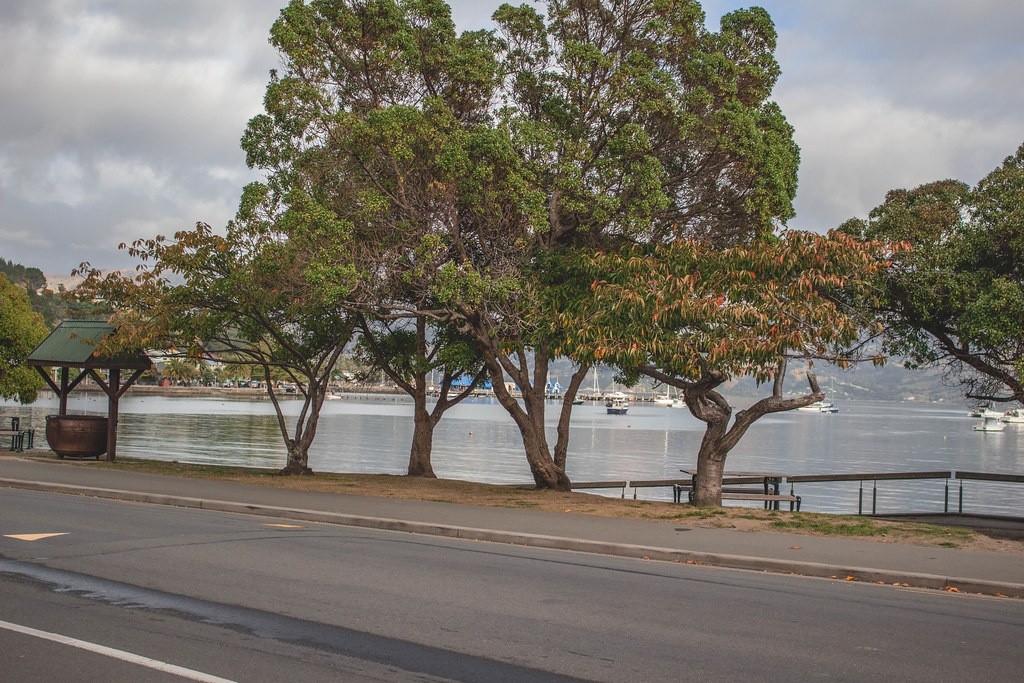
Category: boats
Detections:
[
  {"x1": 966, "y1": 400, "x2": 1024, "y2": 433},
  {"x1": 604, "y1": 394, "x2": 629, "y2": 415},
  {"x1": 796, "y1": 400, "x2": 839, "y2": 414}
]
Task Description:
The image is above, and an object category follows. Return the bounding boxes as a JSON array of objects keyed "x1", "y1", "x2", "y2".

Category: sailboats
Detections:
[{"x1": 572, "y1": 366, "x2": 687, "y2": 409}]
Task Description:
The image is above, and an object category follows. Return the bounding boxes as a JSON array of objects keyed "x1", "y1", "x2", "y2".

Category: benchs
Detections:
[
  {"x1": 689, "y1": 492, "x2": 801, "y2": 513},
  {"x1": 0, "y1": 428, "x2": 35, "y2": 452},
  {"x1": 673, "y1": 484, "x2": 773, "y2": 509}
]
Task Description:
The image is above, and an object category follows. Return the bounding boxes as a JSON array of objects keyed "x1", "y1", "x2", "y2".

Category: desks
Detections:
[
  {"x1": 0, "y1": 414, "x2": 20, "y2": 450},
  {"x1": 679, "y1": 469, "x2": 786, "y2": 510}
]
